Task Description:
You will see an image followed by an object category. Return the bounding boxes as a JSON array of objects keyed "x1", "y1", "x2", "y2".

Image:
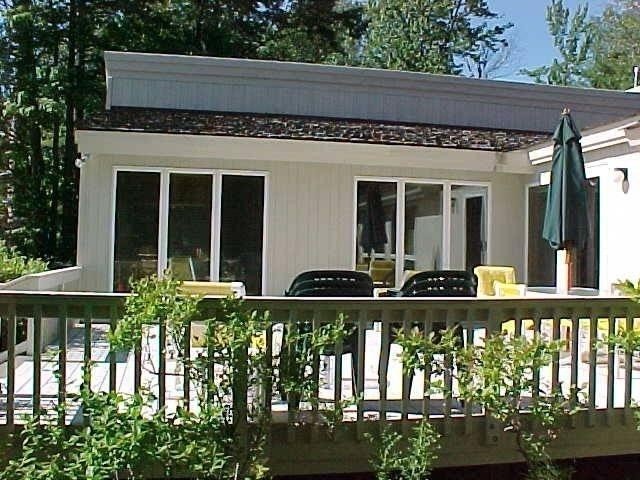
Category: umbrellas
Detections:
[{"x1": 542, "y1": 107, "x2": 589, "y2": 353}]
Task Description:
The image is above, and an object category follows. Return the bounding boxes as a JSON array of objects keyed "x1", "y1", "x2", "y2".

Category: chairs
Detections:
[
  {"x1": 167, "y1": 254, "x2": 196, "y2": 284},
  {"x1": 277, "y1": 269, "x2": 373, "y2": 397},
  {"x1": 166, "y1": 280, "x2": 265, "y2": 349},
  {"x1": 376, "y1": 270, "x2": 478, "y2": 390},
  {"x1": 474, "y1": 265, "x2": 537, "y2": 339},
  {"x1": 368, "y1": 260, "x2": 395, "y2": 284},
  {"x1": 493, "y1": 279, "x2": 528, "y2": 299}
]
